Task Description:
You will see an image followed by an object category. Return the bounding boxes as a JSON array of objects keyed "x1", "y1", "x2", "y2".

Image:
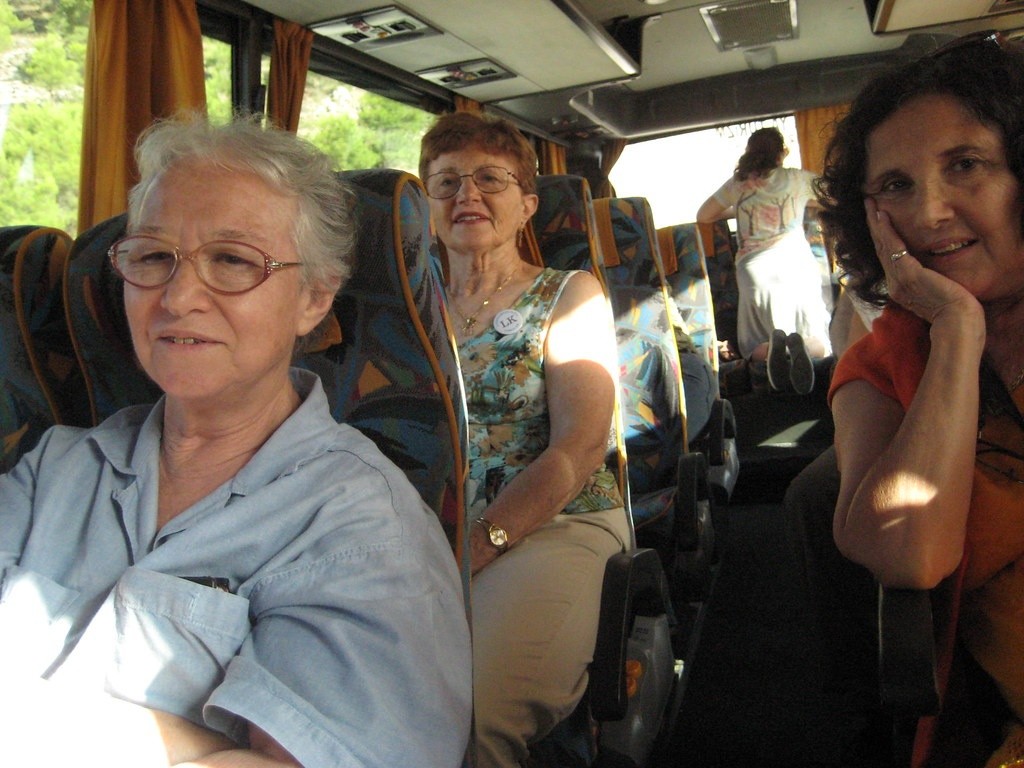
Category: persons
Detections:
[
  {"x1": 419, "y1": 109, "x2": 633, "y2": 768},
  {"x1": 0, "y1": 106, "x2": 477, "y2": 768},
  {"x1": 662, "y1": 271, "x2": 719, "y2": 447},
  {"x1": 808, "y1": 31, "x2": 1023, "y2": 768},
  {"x1": 693, "y1": 127, "x2": 840, "y2": 395}
]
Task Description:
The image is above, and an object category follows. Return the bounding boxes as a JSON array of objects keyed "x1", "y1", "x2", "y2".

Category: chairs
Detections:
[{"x1": 0, "y1": 173, "x2": 1024, "y2": 768}]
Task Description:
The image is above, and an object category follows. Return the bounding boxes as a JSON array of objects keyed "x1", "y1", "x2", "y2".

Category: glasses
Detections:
[
  {"x1": 107, "y1": 236, "x2": 304, "y2": 296},
  {"x1": 424, "y1": 165, "x2": 523, "y2": 199},
  {"x1": 781, "y1": 148, "x2": 788, "y2": 155}
]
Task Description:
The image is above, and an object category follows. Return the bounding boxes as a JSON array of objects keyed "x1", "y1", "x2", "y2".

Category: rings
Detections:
[{"x1": 890, "y1": 249, "x2": 910, "y2": 261}]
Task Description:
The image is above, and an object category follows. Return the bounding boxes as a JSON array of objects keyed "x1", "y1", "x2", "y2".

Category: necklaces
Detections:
[
  {"x1": 1005, "y1": 368, "x2": 1024, "y2": 395},
  {"x1": 446, "y1": 266, "x2": 514, "y2": 335}
]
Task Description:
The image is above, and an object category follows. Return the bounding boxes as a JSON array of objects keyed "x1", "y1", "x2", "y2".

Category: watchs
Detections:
[{"x1": 473, "y1": 516, "x2": 510, "y2": 557}]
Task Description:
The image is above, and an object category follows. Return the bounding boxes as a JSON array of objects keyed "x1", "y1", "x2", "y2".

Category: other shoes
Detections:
[
  {"x1": 788, "y1": 331, "x2": 815, "y2": 396},
  {"x1": 764, "y1": 329, "x2": 789, "y2": 392}
]
